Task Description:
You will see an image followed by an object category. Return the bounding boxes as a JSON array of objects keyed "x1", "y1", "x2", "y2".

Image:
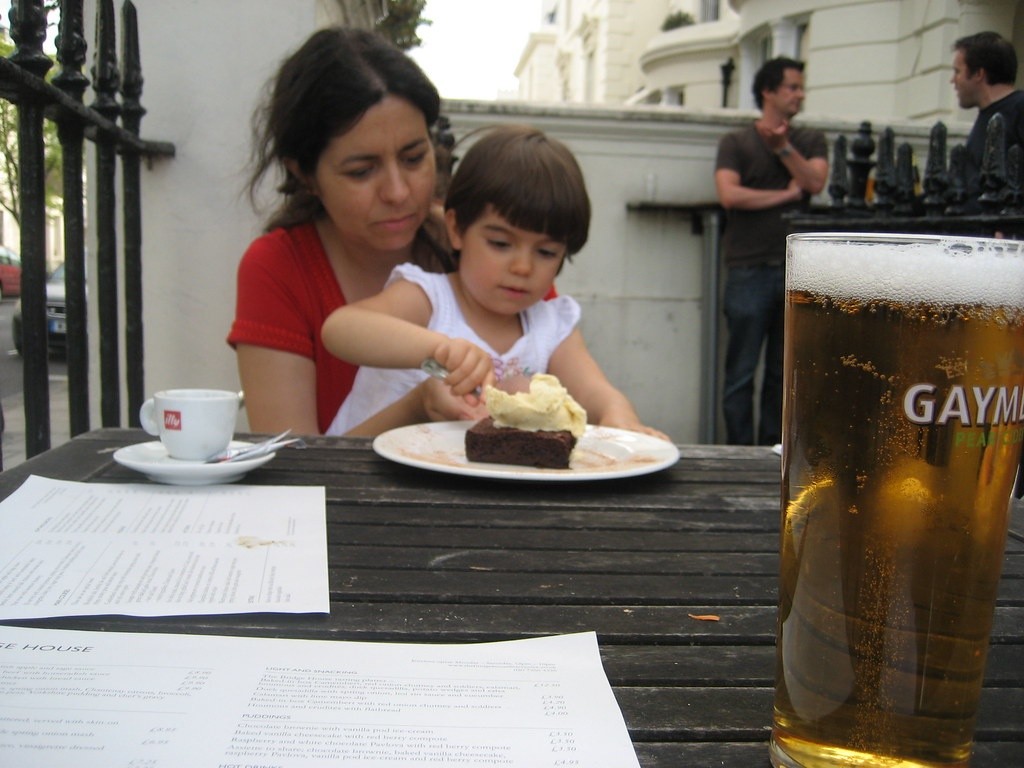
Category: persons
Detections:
[
  {"x1": 321, "y1": 129, "x2": 672, "y2": 444},
  {"x1": 714, "y1": 56, "x2": 829, "y2": 446},
  {"x1": 227, "y1": 26, "x2": 558, "y2": 437},
  {"x1": 950, "y1": 32, "x2": 1024, "y2": 238}
]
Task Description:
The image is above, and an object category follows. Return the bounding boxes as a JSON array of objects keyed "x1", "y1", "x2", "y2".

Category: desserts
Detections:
[{"x1": 464, "y1": 373, "x2": 589, "y2": 470}]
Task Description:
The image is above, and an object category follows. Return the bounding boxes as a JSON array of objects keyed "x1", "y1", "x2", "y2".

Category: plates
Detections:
[
  {"x1": 113, "y1": 439, "x2": 276, "y2": 486},
  {"x1": 372, "y1": 418, "x2": 681, "y2": 482}
]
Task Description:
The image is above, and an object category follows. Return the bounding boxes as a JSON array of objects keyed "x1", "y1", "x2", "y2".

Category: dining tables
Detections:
[{"x1": 0, "y1": 421, "x2": 1024, "y2": 768}]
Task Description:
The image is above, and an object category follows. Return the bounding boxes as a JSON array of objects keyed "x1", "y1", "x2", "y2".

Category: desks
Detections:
[{"x1": 781, "y1": 201, "x2": 1024, "y2": 239}]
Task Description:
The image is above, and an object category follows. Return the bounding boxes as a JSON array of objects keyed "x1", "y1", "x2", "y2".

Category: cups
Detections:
[
  {"x1": 769, "y1": 230, "x2": 1024, "y2": 768},
  {"x1": 139, "y1": 389, "x2": 239, "y2": 462}
]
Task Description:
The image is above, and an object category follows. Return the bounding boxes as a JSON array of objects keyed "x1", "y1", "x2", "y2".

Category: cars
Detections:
[
  {"x1": 11, "y1": 251, "x2": 90, "y2": 359},
  {"x1": 0, "y1": 246, "x2": 22, "y2": 297}
]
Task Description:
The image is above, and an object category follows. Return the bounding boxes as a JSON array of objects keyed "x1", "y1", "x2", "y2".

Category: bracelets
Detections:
[{"x1": 774, "y1": 144, "x2": 793, "y2": 160}]
